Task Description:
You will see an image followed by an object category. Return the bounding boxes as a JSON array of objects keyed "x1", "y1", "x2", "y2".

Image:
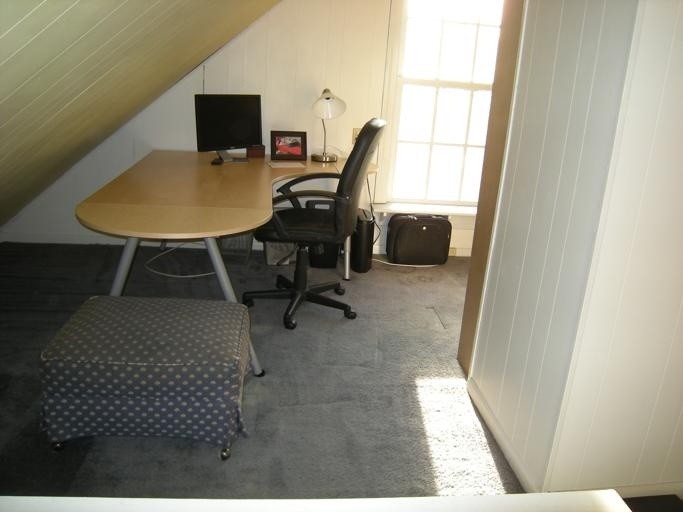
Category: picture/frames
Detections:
[{"x1": 270, "y1": 130, "x2": 307, "y2": 161}]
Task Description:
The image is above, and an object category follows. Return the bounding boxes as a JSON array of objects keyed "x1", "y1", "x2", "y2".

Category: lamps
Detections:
[{"x1": 310, "y1": 89, "x2": 347, "y2": 163}]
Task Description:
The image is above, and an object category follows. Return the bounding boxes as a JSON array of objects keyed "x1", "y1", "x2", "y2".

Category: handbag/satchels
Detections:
[{"x1": 386, "y1": 212, "x2": 452, "y2": 265}]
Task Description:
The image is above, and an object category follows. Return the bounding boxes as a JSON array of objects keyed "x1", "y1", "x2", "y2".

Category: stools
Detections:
[{"x1": 39, "y1": 295, "x2": 252, "y2": 458}]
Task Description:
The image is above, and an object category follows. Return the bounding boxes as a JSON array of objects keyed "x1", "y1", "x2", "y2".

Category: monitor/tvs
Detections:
[{"x1": 194, "y1": 93, "x2": 263, "y2": 163}]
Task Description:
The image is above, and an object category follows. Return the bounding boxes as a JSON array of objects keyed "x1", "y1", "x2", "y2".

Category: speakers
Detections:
[
  {"x1": 309, "y1": 242, "x2": 338, "y2": 268},
  {"x1": 350, "y1": 208, "x2": 375, "y2": 273}
]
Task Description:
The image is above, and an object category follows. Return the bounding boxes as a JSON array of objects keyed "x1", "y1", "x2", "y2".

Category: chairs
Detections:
[{"x1": 242, "y1": 117, "x2": 386, "y2": 329}]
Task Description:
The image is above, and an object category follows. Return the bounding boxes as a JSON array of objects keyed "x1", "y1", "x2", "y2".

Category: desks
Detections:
[{"x1": 73, "y1": 149, "x2": 378, "y2": 377}]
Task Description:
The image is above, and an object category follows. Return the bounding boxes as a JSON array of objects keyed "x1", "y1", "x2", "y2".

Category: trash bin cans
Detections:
[{"x1": 265, "y1": 207, "x2": 299, "y2": 268}]
